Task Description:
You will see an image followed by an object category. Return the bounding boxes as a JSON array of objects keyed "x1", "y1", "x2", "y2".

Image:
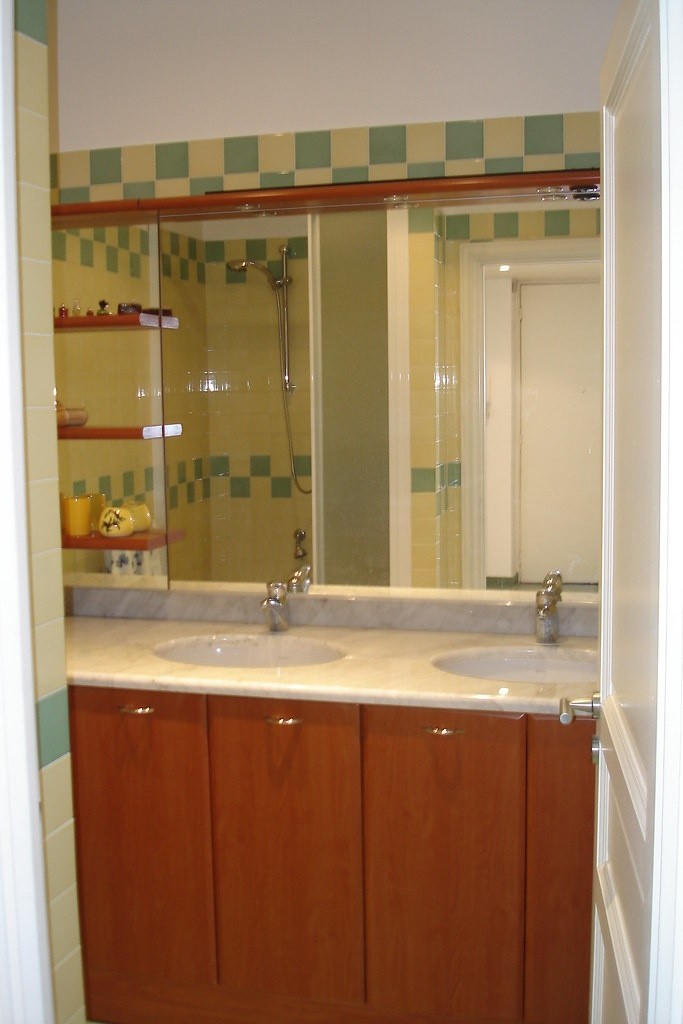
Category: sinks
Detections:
[
  {"x1": 150, "y1": 634, "x2": 349, "y2": 669},
  {"x1": 430, "y1": 646, "x2": 597, "y2": 683}
]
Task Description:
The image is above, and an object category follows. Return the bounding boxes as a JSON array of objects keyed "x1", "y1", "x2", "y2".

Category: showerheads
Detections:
[{"x1": 226, "y1": 259, "x2": 278, "y2": 288}]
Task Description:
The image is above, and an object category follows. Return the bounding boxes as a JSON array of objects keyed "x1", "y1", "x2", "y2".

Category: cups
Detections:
[
  {"x1": 98, "y1": 507, "x2": 134, "y2": 537},
  {"x1": 61, "y1": 494, "x2": 90, "y2": 537},
  {"x1": 121, "y1": 501, "x2": 150, "y2": 532},
  {"x1": 84, "y1": 492, "x2": 106, "y2": 532}
]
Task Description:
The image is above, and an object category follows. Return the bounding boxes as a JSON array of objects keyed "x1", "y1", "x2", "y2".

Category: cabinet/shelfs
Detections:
[
  {"x1": 53, "y1": 312, "x2": 161, "y2": 552},
  {"x1": 359, "y1": 703, "x2": 596, "y2": 1024},
  {"x1": 162, "y1": 317, "x2": 187, "y2": 550},
  {"x1": 67, "y1": 685, "x2": 367, "y2": 1024}
]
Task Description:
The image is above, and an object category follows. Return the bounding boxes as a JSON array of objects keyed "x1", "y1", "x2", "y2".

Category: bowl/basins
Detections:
[{"x1": 57, "y1": 408, "x2": 87, "y2": 427}]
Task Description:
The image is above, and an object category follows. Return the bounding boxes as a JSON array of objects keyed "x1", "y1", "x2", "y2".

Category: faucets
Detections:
[
  {"x1": 535, "y1": 590, "x2": 562, "y2": 642},
  {"x1": 540, "y1": 569, "x2": 564, "y2": 592},
  {"x1": 259, "y1": 582, "x2": 291, "y2": 632},
  {"x1": 286, "y1": 564, "x2": 312, "y2": 593}
]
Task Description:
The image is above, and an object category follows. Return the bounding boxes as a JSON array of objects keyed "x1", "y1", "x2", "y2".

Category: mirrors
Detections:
[{"x1": 52, "y1": 167, "x2": 603, "y2": 607}]
